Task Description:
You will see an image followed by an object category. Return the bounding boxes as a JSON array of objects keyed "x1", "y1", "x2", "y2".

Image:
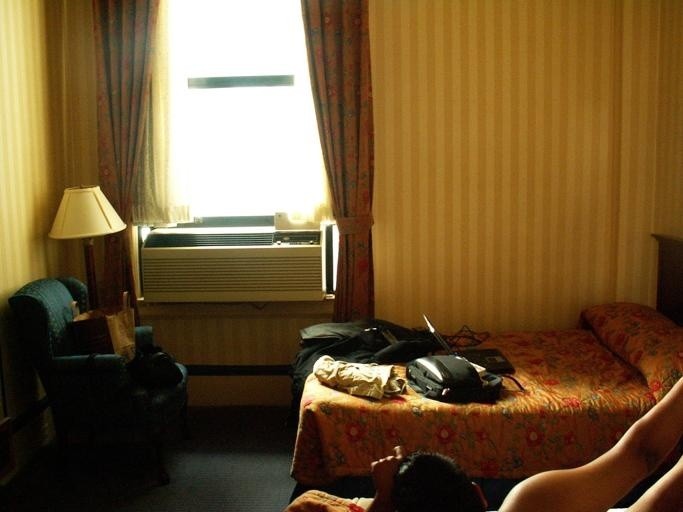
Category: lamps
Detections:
[{"x1": 47, "y1": 184, "x2": 127, "y2": 305}]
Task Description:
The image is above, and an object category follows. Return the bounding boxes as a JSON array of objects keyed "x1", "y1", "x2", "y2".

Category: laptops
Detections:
[{"x1": 422, "y1": 312, "x2": 515, "y2": 374}]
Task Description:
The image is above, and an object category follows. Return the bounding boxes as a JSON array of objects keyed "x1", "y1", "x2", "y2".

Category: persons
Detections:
[{"x1": 366, "y1": 375, "x2": 683, "y2": 512}]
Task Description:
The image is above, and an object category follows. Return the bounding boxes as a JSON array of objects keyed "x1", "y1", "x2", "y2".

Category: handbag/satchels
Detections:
[
  {"x1": 71, "y1": 291, "x2": 137, "y2": 365},
  {"x1": 408, "y1": 353, "x2": 504, "y2": 404}
]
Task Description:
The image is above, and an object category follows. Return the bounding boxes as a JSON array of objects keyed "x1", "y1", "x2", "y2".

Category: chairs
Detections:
[{"x1": 7, "y1": 276, "x2": 188, "y2": 486}]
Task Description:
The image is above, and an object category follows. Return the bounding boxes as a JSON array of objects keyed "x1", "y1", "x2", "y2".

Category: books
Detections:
[{"x1": 469, "y1": 362, "x2": 488, "y2": 378}]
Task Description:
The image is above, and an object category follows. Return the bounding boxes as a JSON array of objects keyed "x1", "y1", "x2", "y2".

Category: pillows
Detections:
[{"x1": 579, "y1": 300, "x2": 681, "y2": 403}]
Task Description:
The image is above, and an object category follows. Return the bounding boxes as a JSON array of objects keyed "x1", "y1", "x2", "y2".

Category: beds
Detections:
[{"x1": 290, "y1": 234, "x2": 683, "y2": 486}]
